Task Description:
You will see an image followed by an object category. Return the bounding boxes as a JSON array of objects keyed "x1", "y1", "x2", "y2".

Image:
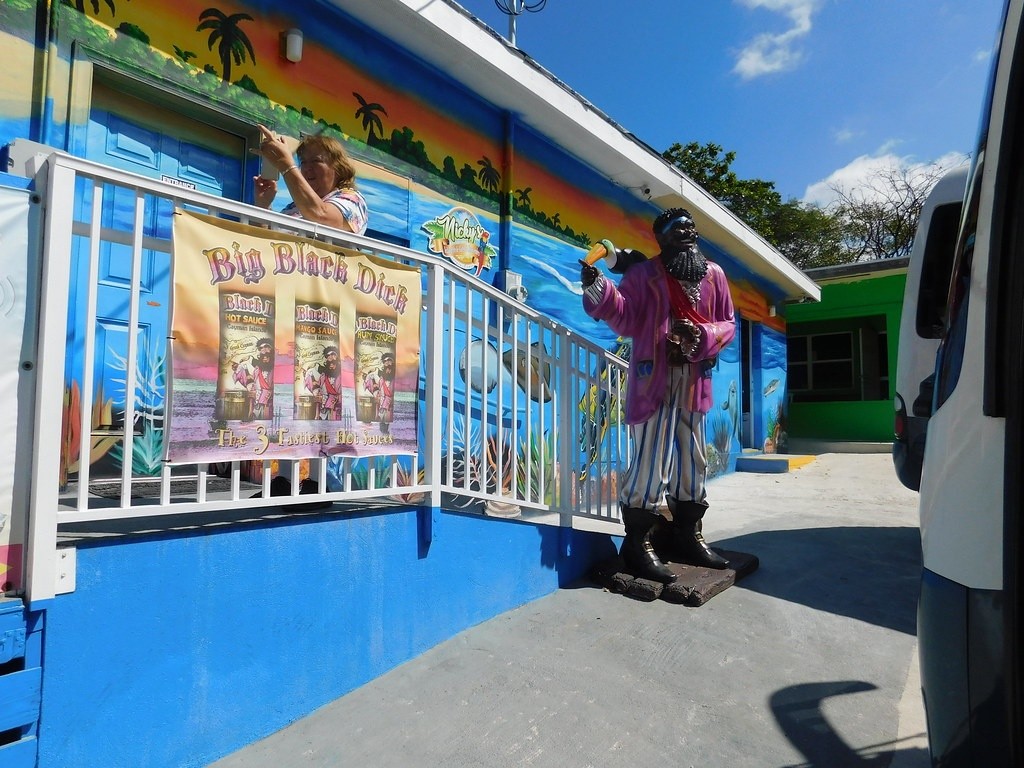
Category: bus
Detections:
[
  {"x1": 915, "y1": 0, "x2": 1024, "y2": 765},
  {"x1": 888, "y1": 168, "x2": 968, "y2": 488}
]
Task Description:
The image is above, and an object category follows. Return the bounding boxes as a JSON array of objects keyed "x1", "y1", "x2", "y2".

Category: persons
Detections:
[
  {"x1": 578, "y1": 207, "x2": 737, "y2": 586},
  {"x1": 249, "y1": 122, "x2": 371, "y2": 512}
]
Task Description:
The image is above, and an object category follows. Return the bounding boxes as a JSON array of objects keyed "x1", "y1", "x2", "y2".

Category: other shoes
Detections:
[
  {"x1": 284, "y1": 480, "x2": 332, "y2": 511},
  {"x1": 250, "y1": 476, "x2": 292, "y2": 497}
]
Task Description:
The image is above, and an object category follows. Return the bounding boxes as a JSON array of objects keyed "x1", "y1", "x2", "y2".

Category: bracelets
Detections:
[{"x1": 281, "y1": 165, "x2": 297, "y2": 177}]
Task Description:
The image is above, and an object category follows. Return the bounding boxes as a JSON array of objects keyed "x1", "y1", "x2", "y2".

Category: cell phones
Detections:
[{"x1": 261, "y1": 138, "x2": 279, "y2": 181}]
